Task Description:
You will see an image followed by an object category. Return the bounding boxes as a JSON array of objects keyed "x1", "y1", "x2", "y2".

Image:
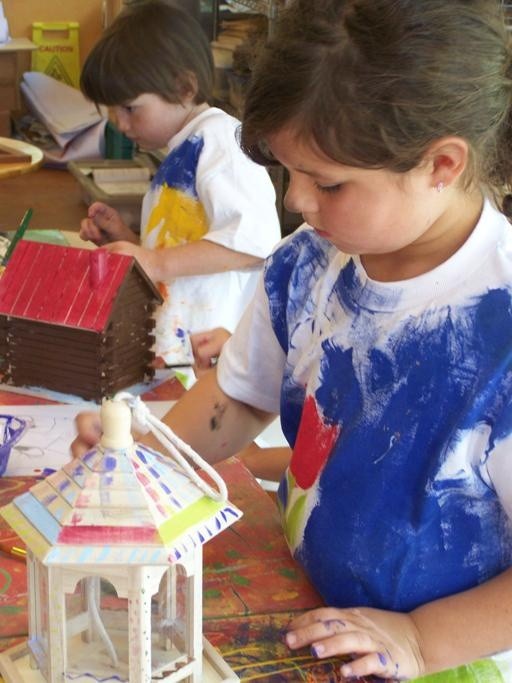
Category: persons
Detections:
[
  {"x1": 77, "y1": 0, "x2": 297, "y2": 495},
  {"x1": 189, "y1": 325, "x2": 293, "y2": 485},
  {"x1": 68, "y1": 0, "x2": 512, "y2": 682}
]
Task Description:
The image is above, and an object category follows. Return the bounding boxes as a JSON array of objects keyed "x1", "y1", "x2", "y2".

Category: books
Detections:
[{"x1": 20, "y1": 69, "x2": 169, "y2": 209}]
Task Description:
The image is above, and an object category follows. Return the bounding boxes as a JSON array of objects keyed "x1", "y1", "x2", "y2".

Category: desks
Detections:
[{"x1": 0, "y1": 166, "x2": 401, "y2": 683}]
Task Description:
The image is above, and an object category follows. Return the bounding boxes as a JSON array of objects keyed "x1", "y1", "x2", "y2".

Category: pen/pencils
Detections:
[
  {"x1": 0, "y1": 542, "x2": 27, "y2": 558},
  {"x1": 0, "y1": 207, "x2": 32, "y2": 267},
  {"x1": 153, "y1": 360, "x2": 215, "y2": 366}
]
